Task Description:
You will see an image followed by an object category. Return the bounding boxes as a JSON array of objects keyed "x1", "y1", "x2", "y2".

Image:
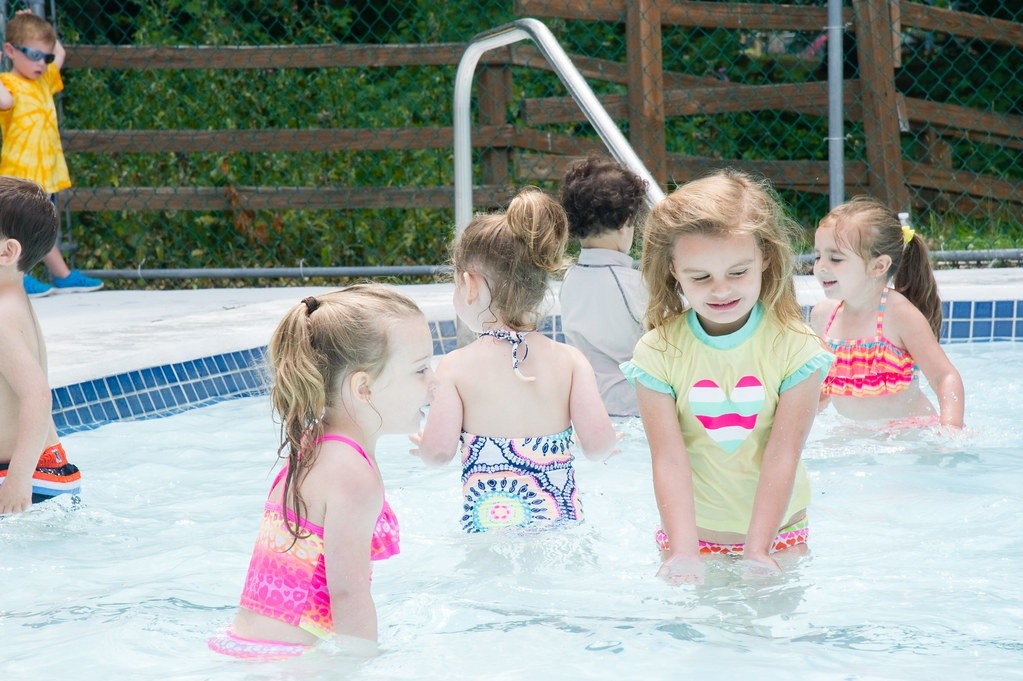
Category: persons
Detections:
[
  {"x1": 222, "y1": 282, "x2": 438, "y2": 661},
  {"x1": 0, "y1": 11, "x2": 106, "y2": 299},
  {"x1": 800, "y1": 194, "x2": 966, "y2": 439},
  {"x1": 558, "y1": 155, "x2": 666, "y2": 415},
  {"x1": 621, "y1": 167, "x2": 840, "y2": 578},
  {"x1": 405, "y1": 190, "x2": 620, "y2": 531},
  {"x1": 0, "y1": 174, "x2": 78, "y2": 518}
]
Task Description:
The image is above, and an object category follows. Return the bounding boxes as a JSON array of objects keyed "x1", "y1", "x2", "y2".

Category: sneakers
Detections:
[
  {"x1": 52, "y1": 268, "x2": 104, "y2": 294},
  {"x1": 24, "y1": 274, "x2": 52, "y2": 297}
]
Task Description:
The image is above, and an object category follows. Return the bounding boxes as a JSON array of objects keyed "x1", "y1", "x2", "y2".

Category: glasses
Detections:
[{"x1": 11, "y1": 41, "x2": 55, "y2": 64}]
